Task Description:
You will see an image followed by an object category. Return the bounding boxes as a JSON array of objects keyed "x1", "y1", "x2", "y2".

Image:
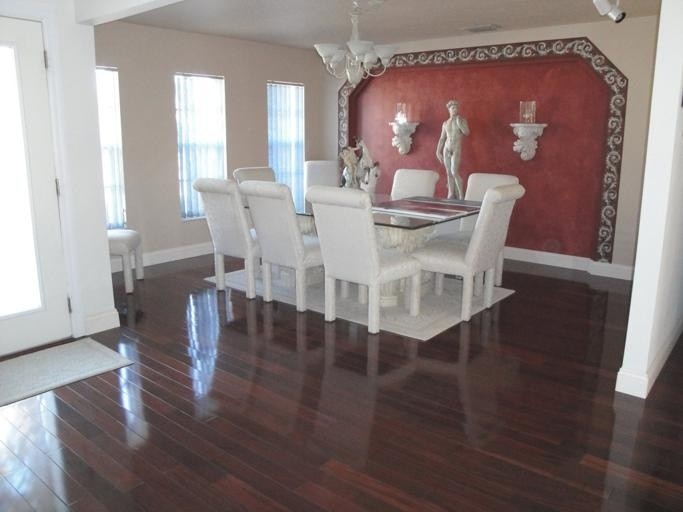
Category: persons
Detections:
[
  {"x1": 434, "y1": 101, "x2": 471, "y2": 201},
  {"x1": 337, "y1": 145, "x2": 364, "y2": 191}
]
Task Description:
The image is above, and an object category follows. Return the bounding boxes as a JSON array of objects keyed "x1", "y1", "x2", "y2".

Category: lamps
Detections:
[{"x1": 312, "y1": 1, "x2": 395, "y2": 90}]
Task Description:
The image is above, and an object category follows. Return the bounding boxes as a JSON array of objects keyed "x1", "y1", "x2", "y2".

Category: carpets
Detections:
[
  {"x1": 0, "y1": 336, "x2": 134, "y2": 407},
  {"x1": 201, "y1": 268, "x2": 516, "y2": 342}
]
